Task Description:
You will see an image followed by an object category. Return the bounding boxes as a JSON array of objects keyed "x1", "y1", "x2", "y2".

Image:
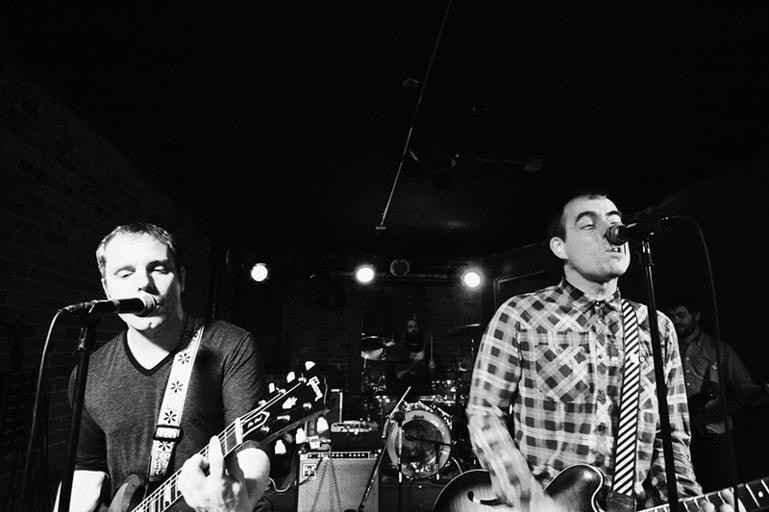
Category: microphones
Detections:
[
  {"x1": 605, "y1": 207, "x2": 683, "y2": 246},
  {"x1": 56, "y1": 293, "x2": 160, "y2": 321}
]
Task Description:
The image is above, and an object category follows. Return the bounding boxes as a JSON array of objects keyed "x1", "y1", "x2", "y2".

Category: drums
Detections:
[
  {"x1": 419, "y1": 394, "x2": 468, "y2": 414},
  {"x1": 386, "y1": 401, "x2": 457, "y2": 481},
  {"x1": 431, "y1": 378, "x2": 471, "y2": 394}
]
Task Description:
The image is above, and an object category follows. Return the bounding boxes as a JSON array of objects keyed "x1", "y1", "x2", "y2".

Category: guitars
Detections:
[
  {"x1": 433, "y1": 463, "x2": 768, "y2": 510},
  {"x1": 106, "y1": 359, "x2": 331, "y2": 512}
]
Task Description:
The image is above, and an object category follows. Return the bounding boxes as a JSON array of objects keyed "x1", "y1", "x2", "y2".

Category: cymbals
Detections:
[
  {"x1": 442, "y1": 367, "x2": 473, "y2": 373},
  {"x1": 448, "y1": 323, "x2": 485, "y2": 332}
]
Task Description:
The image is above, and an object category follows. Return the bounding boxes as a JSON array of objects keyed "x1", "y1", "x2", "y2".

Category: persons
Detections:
[
  {"x1": 53, "y1": 222, "x2": 272, "y2": 512},
  {"x1": 386, "y1": 317, "x2": 442, "y2": 403},
  {"x1": 465, "y1": 189, "x2": 747, "y2": 512},
  {"x1": 662, "y1": 294, "x2": 769, "y2": 495}
]
